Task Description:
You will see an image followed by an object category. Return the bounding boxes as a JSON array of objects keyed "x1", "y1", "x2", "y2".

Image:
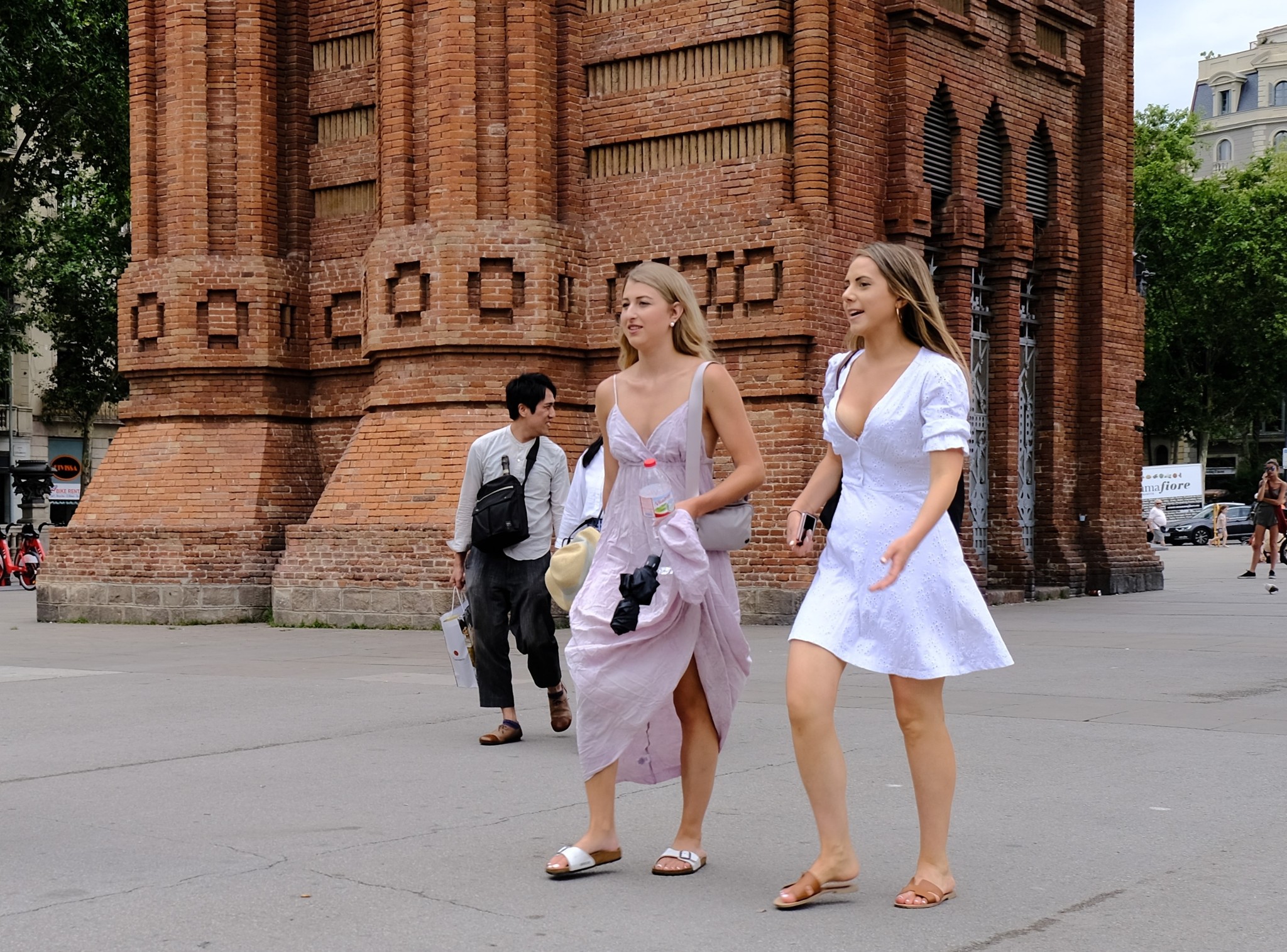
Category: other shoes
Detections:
[
  {"x1": 479, "y1": 719, "x2": 523, "y2": 745},
  {"x1": 1237, "y1": 569, "x2": 1256, "y2": 579},
  {"x1": 1268, "y1": 570, "x2": 1276, "y2": 579},
  {"x1": 1208, "y1": 539, "x2": 1211, "y2": 547},
  {"x1": 547, "y1": 681, "x2": 573, "y2": 732},
  {"x1": 1220, "y1": 545, "x2": 1229, "y2": 548}
]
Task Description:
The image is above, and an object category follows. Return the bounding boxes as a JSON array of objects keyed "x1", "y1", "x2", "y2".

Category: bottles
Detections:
[{"x1": 640, "y1": 459, "x2": 674, "y2": 575}]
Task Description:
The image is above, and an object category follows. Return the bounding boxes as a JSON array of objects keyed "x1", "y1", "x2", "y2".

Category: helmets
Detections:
[{"x1": 1254, "y1": 493, "x2": 1258, "y2": 499}]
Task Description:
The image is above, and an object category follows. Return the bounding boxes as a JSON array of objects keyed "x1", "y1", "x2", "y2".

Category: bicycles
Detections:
[{"x1": 0, "y1": 521, "x2": 55, "y2": 591}]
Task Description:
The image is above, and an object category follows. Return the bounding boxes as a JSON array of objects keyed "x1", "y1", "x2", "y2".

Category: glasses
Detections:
[
  {"x1": 1265, "y1": 466, "x2": 1275, "y2": 471},
  {"x1": 1156, "y1": 502, "x2": 1161, "y2": 505}
]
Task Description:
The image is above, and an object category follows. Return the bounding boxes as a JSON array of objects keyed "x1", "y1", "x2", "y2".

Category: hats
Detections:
[{"x1": 545, "y1": 526, "x2": 600, "y2": 611}]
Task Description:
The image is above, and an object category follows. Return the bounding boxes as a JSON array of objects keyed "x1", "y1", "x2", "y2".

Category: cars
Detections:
[{"x1": 1164, "y1": 501, "x2": 1256, "y2": 547}]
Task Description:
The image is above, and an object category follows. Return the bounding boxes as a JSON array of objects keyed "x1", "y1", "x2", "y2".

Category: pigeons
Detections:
[{"x1": 1262, "y1": 583, "x2": 1279, "y2": 595}]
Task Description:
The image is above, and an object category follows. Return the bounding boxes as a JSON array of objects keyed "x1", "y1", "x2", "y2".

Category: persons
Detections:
[
  {"x1": 1237, "y1": 459, "x2": 1287, "y2": 579},
  {"x1": 446, "y1": 373, "x2": 572, "y2": 746},
  {"x1": 1147, "y1": 499, "x2": 1167, "y2": 547},
  {"x1": 1208, "y1": 504, "x2": 1230, "y2": 548},
  {"x1": 775, "y1": 241, "x2": 1015, "y2": 910},
  {"x1": 552, "y1": 430, "x2": 606, "y2": 554},
  {"x1": 545, "y1": 264, "x2": 767, "y2": 880}
]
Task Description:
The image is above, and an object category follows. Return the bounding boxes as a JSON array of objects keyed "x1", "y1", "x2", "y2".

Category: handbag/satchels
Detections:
[
  {"x1": 694, "y1": 501, "x2": 754, "y2": 551},
  {"x1": 819, "y1": 464, "x2": 965, "y2": 538},
  {"x1": 1275, "y1": 505, "x2": 1287, "y2": 534},
  {"x1": 439, "y1": 585, "x2": 479, "y2": 688},
  {"x1": 1160, "y1": 525, "x2": 1168, "y2": 533},
  {"x1": 471, "y1": 436, "x2": 540, "y2": 555}
]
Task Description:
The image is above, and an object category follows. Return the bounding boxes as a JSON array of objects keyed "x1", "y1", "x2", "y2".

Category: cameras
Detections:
[{"x1": 796, "y1": 511, "x2": 817, "y2": 544}]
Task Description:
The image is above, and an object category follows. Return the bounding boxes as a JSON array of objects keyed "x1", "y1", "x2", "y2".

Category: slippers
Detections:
[
  {"x1": 546, "y1": 845, "x2": 622, "y2": 875},
  {"x1": 652, "y1": 848, "x2": 707, "y2": 875},
  {"x1": 773, "y1": 870, "x2": 859, "y2": 908},
  {"x1": 894, "y1": 876, "x2": 956, "y2": 908}
]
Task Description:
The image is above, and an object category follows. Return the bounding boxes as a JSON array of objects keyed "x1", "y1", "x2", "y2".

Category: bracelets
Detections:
[{"x1": 786, "y1": 510, "x2": 801, "y2": 519}]
